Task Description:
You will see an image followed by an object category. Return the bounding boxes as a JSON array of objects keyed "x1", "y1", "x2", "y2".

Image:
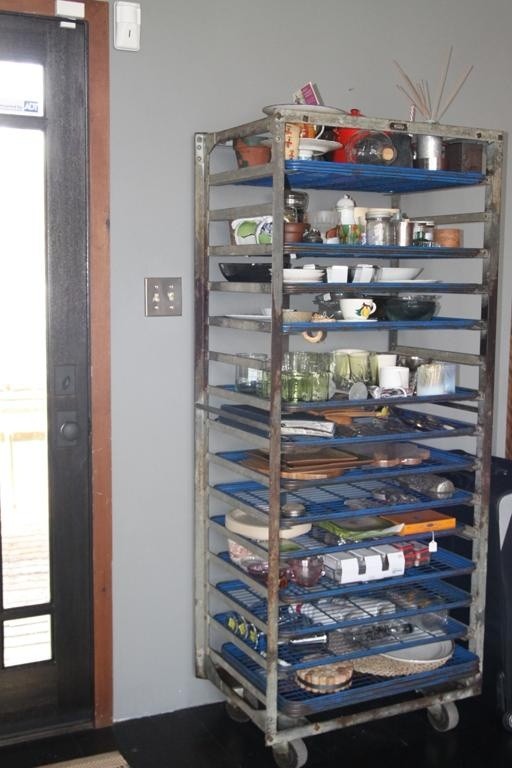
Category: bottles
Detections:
[{"x1": 365, "y1": 210, "x2": 397, "y2": 246}]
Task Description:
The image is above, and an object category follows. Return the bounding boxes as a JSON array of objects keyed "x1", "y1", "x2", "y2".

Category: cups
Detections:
[
  {"x1": 343, "y1": 224, "x2": 362, "y2": 244},
  {"x1": 340, "y1": 299, "x2": 377, "y2": 319},
  {"x1": 289, "y1": 557, "x2": 324, "y2": 586},
  {"x1": 380, "y1": 367, "x2": 410, "y2": 389},
  {"x1": 236, "y1": 353, "x2": 330, "y2": 402},
  {"x1": 231, "y1": 216, "x2": 275, "y2": 245},
  {"x1": 284, "y1": 222, "x2": 304, "y2": 241}
]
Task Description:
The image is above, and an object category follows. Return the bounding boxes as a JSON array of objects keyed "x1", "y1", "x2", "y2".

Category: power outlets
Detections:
[{"x1": 144, "y1": 276, "x2": 182, "y2": 317}]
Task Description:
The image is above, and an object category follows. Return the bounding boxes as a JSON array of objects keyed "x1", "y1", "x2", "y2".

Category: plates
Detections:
[
  {"x1": 375, "y1": 630, "x2": 455, "y2": 664},
  {"x1": 259, "y1": 137, "x2": 344, "y2": 157},
  {"x1": 336, "y1": 318, "x2": 378, "y2": 322},
  {"x1": 372, "y1": 280, "x2": 442, "y2": 283},
  {"x1": 268, "y1": 269, "x2": 325, "y2": 284}
]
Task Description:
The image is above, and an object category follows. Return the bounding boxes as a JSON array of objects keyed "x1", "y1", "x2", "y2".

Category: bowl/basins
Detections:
[
  {"x1": 351, "y1": 268, "x2": 424, "y2": 279},
  {"x1": 218, "y1": 261, "x2": 291, "y2": 282},
  {"x1": 280, "y1": 311, "x2": 313, "y2": 323}
]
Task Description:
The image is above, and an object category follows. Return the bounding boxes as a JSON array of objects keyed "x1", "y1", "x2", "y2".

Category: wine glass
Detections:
[{"x1": 308, "y1": 211, "x2": 341, "y2": 244}]
